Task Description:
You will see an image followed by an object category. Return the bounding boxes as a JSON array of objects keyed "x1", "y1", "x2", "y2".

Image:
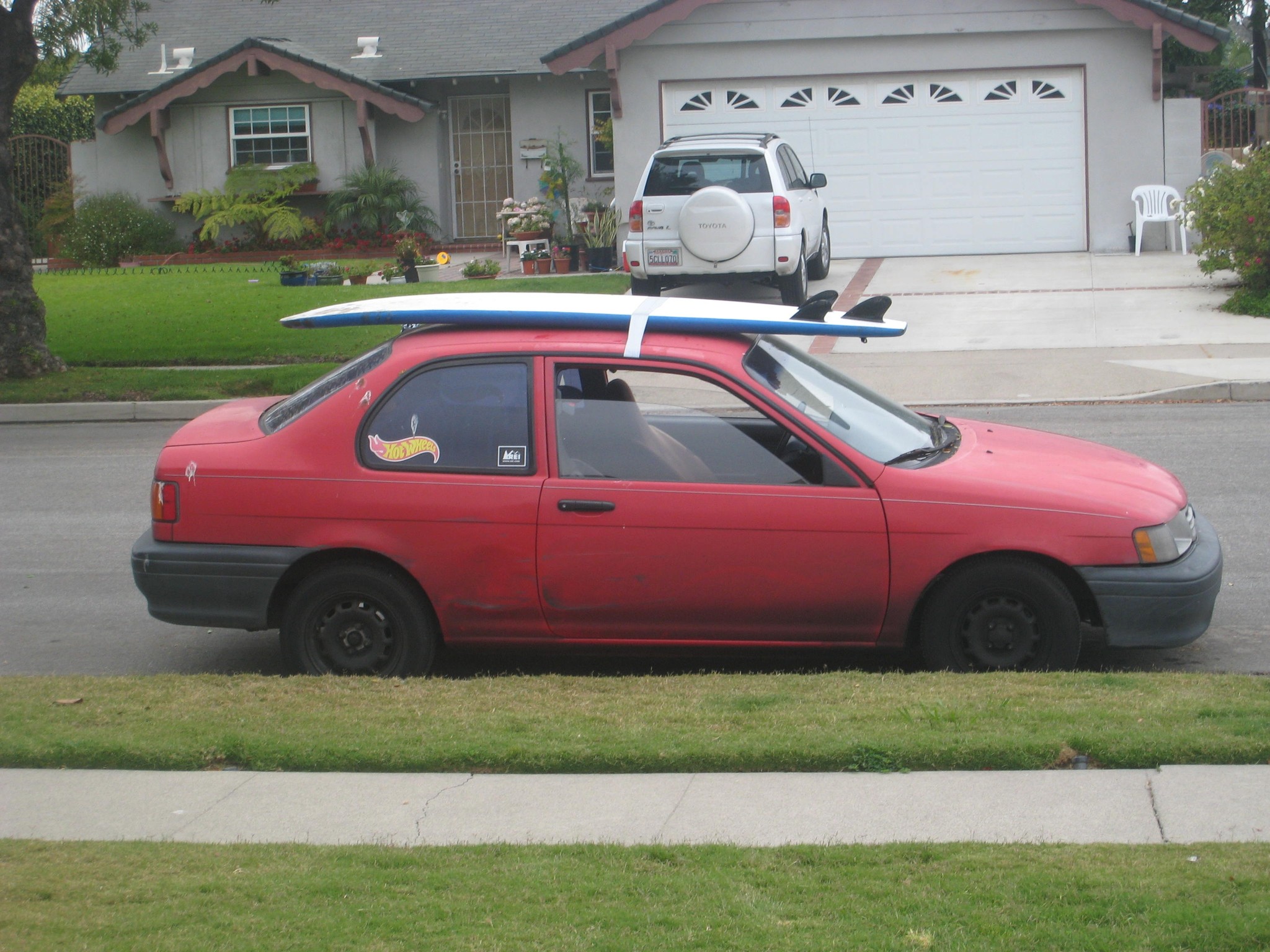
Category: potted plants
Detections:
[
  {"x1": 573, "y1": 201, "x2": 629, "y2": 272},
  {"x1": 287, "y1": 178, "x2": 321, "y2": 192},
  {"x1": 279, "y1": 253, "x2": 344, "y2": 286}
]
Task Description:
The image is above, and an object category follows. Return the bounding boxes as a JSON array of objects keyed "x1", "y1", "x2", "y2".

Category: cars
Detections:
[{"x1": 121, "y1": 323, "x2": 1224, "y2": 675}]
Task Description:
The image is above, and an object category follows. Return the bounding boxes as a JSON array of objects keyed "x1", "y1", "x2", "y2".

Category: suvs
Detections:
[{"x1": 621, "y1": 128, "x2": 834, "y2": 309}]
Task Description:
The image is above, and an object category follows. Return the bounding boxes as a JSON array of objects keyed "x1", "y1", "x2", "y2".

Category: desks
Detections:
[{"x1": 496, "y1": 211, "x2": 538, "y2": 257}]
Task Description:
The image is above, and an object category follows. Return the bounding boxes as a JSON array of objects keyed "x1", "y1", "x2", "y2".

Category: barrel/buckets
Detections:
[{"x1": 1128, "y1": 236, "x2": 1142, "y2": 252}]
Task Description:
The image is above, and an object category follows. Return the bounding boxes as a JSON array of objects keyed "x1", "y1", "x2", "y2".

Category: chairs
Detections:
[
  {"x1": 1131, "y1": 185, "x2": 1186, "y2": 256},
  {"x1": 668, "y1": 157, "x2": 772, "y2": 196},
  {"x1": 555, "y1": 380, "x2": 717, "y2": 483}
]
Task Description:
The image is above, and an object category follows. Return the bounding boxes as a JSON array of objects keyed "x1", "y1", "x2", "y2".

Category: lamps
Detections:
[
  {"x1": 350, "y1": 37, "x2": 383, "y2": 59},
  {"x1": 167, "y1": 47, "x2": 195, "y2": 70}
]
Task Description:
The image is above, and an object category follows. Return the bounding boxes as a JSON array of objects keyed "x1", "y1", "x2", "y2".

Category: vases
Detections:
[
  {"x1": 348, "y1": 257, "x2": 439, "y2": 285},
  {"x1": 509, "y1": 231, "x2": 569, "y2": 274},
  {"x1": 464, "y1": 271, "x2": 500, "y2": 280}
]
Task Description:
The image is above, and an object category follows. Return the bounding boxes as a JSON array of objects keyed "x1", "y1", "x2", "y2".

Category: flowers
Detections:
[
  {"x1": 500, "y1": 196, "x2": 570, "y2": 259},
  {"x1": 345, "y1": 233, "x2": 436, "y2": 282},
  {"x1": 459, "y1": 256, "x2": 501, "y2": 278}
]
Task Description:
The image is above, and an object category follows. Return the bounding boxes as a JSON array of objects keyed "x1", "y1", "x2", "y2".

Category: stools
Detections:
[{"x1": 506, "y1": 238, "x2": 553, "y2": 274}]
